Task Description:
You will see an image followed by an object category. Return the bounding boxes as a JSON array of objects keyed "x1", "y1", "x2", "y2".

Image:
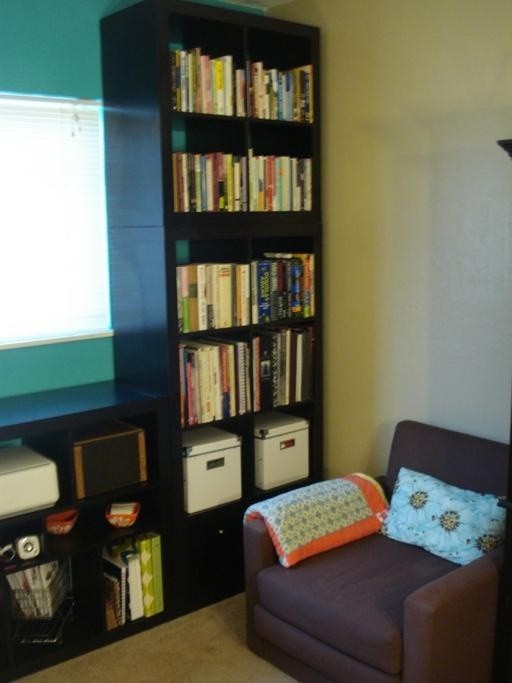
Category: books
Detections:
[
  {"x1": 101, "y1": 531, "x2": 164, "y2": 631},
  {"x1": 6, "y1": 556, "x2": 72, "y2": 620},
  {"x1": 171, "y1": 48, "x2": 314, "y2": 124},
  {"x1": 176, "y1": 253, "x2": 315, "y2": 428},
  {"x1": 172, "y1": 148, "x2": 312, "y2": 212}
]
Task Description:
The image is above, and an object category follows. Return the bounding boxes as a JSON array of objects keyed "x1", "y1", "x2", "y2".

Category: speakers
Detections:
[{"x1": 15, "y1": 534, "x2": 40, "y2": 561}]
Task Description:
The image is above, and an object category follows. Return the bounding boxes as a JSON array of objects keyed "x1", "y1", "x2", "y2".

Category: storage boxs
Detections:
[
  {"x1": 182, "y1": 424, "x2": 244, "y2": 512},
  {"x1": 65, "y1": 426, "x2": 148, "y2": 497},
  {"x1": 251, "y1": 417, "x2": 311, "y2": 493},
  {"x1": 4, "y1": 443, "x2": 59, "y2": 522}
]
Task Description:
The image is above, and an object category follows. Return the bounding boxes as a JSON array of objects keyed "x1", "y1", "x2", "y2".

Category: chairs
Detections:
[{"x1": 243, "y1": 417, "x2": 512, "y2": 683}]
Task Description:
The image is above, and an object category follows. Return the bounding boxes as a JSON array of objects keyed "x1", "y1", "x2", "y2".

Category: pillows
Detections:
[{"x1": 381, "y1": 467, "x2": 507, "y2": 568}]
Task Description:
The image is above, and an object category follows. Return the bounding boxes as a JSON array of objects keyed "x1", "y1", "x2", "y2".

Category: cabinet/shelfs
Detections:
[
  {"x1": 4, "y1": 376, "x2": 184, "y2": 679},
  {"x1": 100, "y1": 5, "x2": 325, "y2": 616}
]
